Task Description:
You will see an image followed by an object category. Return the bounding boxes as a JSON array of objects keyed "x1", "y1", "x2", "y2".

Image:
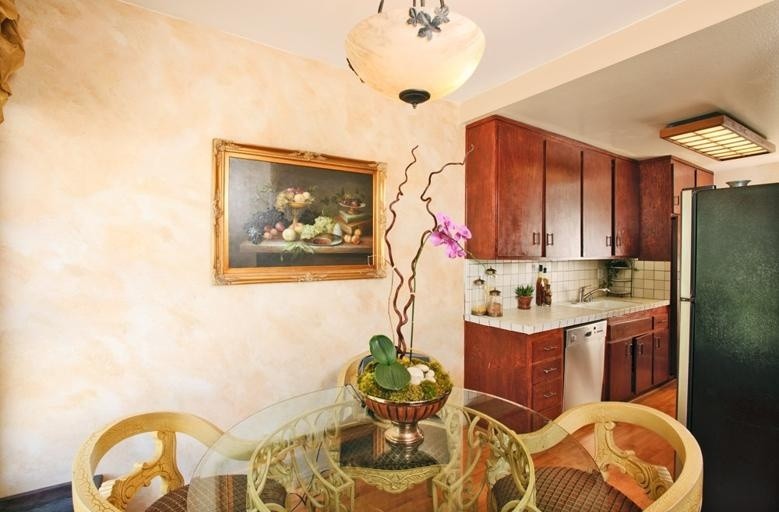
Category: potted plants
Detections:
[{"x1": 357, "y1": 353, "x2": 454, "y2": 449}]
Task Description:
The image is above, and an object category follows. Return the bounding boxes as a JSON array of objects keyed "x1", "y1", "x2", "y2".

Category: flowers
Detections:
[{"x1": 358, "y1": 143, "x2": 471, "y2": 403}]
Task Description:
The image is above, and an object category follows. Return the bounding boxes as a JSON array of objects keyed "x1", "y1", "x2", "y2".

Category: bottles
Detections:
[
  {"x1": 535, "y1": 264, "x2": 553, "y2": 308},
  {"x1": 470, "y1": 264, "x2": 503, "y2": 318}
]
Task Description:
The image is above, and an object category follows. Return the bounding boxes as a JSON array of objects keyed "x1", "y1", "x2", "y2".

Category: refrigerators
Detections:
[{"x1": 676, "y1": 182, "x2": 779, "y2": 512}]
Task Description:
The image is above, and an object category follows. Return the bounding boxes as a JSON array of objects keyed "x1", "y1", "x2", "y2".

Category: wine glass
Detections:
[{"x1": 359, "y1": 384, "x2": 453, "y2": 446}]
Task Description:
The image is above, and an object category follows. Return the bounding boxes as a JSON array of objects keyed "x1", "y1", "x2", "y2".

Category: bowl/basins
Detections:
[{"x1": 725, "y1": 179, "x2": 753, "y2": 188}]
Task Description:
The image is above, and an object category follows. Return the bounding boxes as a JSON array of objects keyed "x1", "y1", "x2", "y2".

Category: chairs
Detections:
[
  {"x1": 72, "y1": 410, "x2": 315, "y2": 512},
  {"x1": 325, "y1": 350, "x2": 463, "y2": 511},
  {"x1": 484, "y1": 401, "x2": 703, "y2": 512}
]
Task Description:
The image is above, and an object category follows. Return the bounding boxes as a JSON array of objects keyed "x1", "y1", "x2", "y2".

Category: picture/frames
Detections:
[{"x1": 211, "y1": 138, "x2": 388, "y2": 286}]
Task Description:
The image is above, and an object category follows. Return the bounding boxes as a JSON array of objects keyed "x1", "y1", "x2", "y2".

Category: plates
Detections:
[{"x1": 309, "y1": 235, "x2": 343, "y2": 246}]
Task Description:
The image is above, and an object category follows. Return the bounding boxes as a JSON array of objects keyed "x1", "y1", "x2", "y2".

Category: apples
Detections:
[
  {"x1": 294, "y1": 194, "x2": 305, "y2": 202},
  {"x1": 343, "y1": 228, "x2": 362, "y2": 245},
  {"x1": 263, "y1": 222, "x2": 303, "y2": 241}
]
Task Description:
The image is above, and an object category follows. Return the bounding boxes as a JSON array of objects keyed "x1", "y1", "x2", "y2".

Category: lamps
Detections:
[
  {"x1": 658, "y1": 113, "x2": 776, "y2": 162},
  {"x1": 345, "y1": 0, "x2": 487, "y2": 109}
]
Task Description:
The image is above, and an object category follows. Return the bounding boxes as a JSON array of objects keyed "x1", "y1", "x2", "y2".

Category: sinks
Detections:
[{"x1": 569, "y1": 299, "x2": 641, "y2": 312}]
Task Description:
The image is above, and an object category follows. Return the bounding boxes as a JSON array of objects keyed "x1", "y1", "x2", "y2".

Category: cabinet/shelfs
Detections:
[
  {"x1": 464, "y1": 296, "x2": 675, "y2": 433},
  {"x1": 465, "y1": 115, "x2": 713, "y2": 261}
]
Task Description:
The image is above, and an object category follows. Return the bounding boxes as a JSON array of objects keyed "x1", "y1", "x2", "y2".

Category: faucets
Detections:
[{"x1": 578, "y1": 284, "x2": 610, "y2": 300}]
